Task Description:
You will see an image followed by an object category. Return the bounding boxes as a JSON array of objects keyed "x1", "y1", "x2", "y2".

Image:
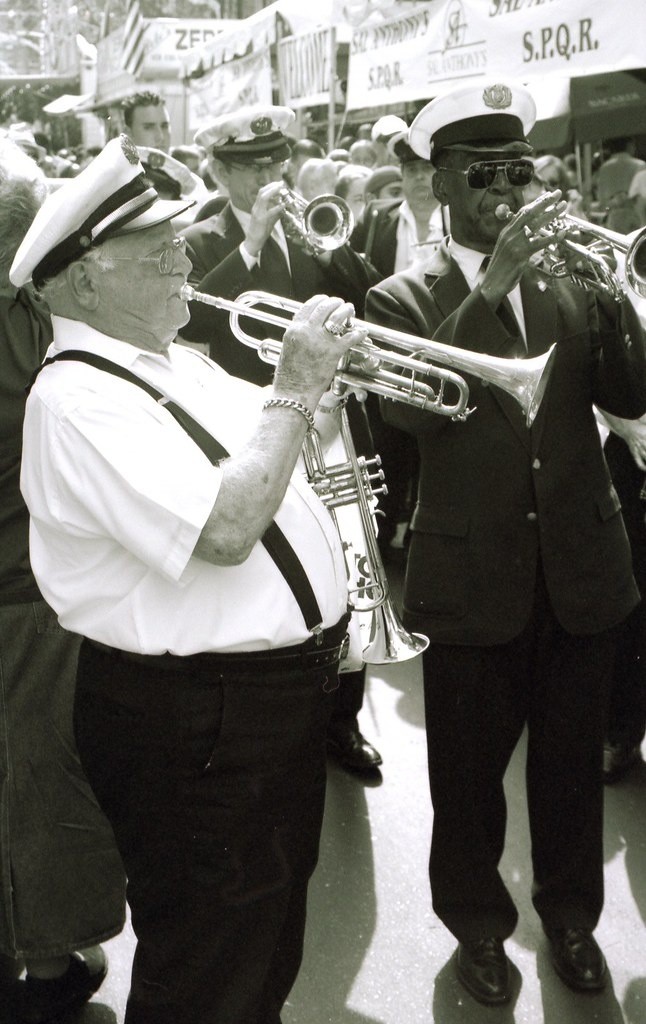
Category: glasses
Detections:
[
  {"x1": 94, "y1": 235, "x2": 186, "y2": 273},
  {"x1": 226, "y1": 164, "x2": 285, "y2": 176},
  {"x1": 435, "y1": 160, "x2": 535, "y2": 192}
]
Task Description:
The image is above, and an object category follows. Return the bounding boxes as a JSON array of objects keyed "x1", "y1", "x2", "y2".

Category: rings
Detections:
[
  {"x1": 524, "y1": 225, "x2": 533, "y2": 238},
  {"x1": 326, "y1": 321, "x2": 344, "y2": 335}
]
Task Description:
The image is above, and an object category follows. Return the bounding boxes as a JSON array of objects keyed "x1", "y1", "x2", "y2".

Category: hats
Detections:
[
  {"x1": 364, "y1": 166, "x2": 403, "y2": 192},
  {"x1": 9, "y1": 134, "x2": 197, "y2": 289},
  {"x1": 195, "y1": 104, "x2": 295, "y2": 167},
  {"x1": 136, "y1": 145, "x2": 195, "y2": 203},
  {"x1": 371, "y1": 114, "x2": 408, "y2": 142},
  {"x1": 388, "y1": 132, "x2": 426, "y2": 164},
  {"x1": 408, "y1": 77, "x2": 536, "y2": 163}
]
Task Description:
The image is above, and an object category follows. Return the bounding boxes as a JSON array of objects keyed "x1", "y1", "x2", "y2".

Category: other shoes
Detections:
[{"x1": 21, "y1": 938, "x2": 108, "y2": 1015}]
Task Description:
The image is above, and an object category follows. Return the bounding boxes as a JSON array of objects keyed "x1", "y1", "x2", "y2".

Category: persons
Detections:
[{"x1": 0, "y1": 84, "x2": 646, "y2": 1024}]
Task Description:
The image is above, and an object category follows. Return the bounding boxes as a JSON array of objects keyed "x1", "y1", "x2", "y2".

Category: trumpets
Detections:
[
  {"x1": 275, "y1": 188, "x2": 358, "y2": 252},
  {"x1": 302, "y1": 401, "x2": 431, "y2": 665},
  {"x1": 179, "y1": 281, "x2": 560, "y2": 430},
  {"x1": 493, "y1": 199, "x2": 646, "y2": 300}
]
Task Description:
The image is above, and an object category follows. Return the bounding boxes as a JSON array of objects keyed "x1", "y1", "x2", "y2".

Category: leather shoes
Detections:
[
  {"x1": 324, "y1": 719, "x2": 381, "y2": 770},
  {"x1": 541, "y1": 914, "x2": 611, "y2": 995},
  {"x1": 601, "y1": 739, "x2": 640, "y2": 786},
  {"x1": 459, "y1": 937, "x2": 514, "y2": 1007}
]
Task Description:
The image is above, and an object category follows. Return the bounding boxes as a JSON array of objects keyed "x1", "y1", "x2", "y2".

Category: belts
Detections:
[{"x1": 91, "y1": 637, "x2": 346, "y2": 677}]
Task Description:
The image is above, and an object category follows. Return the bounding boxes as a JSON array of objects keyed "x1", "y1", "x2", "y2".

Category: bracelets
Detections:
[{"x1": 264, "y1": 398, "x2": 314, "y2": 435}]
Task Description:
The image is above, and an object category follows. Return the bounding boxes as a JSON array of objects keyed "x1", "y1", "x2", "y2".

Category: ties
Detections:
[
  {"x1": 482, "y1": 256, "x2": 526, "y2": 354},
  {"x1": 261, "y1": 237, "x2": 292, "y2": 299}
]
